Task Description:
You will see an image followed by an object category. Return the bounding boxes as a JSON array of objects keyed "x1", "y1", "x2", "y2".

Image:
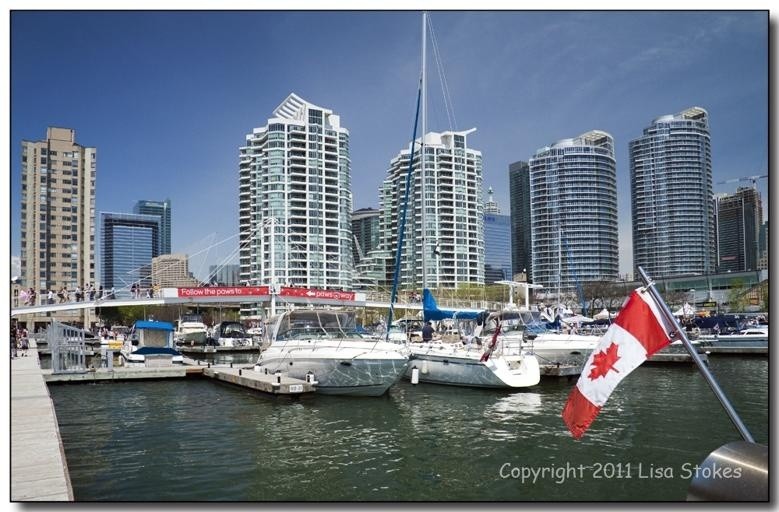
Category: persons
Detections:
[
  {"x1": 213, "y1": 280, "x2": 218, "y2": 287},
  {"x1": 94, "y1": 328, "x2": 129, "y2": 341},
  {"x1": 209, "y1": 281, "x2": 214, "y2": 288},
  {"x1": 10, "y1": 320, "x2": 29, "y2": 359},
  {"x1": 422, "y1": 322, "x2": 434, "y2": 341},
  {"x1": 20, "y1": 282, "x2": 161, "y2": 306}
]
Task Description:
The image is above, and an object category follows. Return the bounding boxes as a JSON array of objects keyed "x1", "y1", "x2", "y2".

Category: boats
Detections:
[
  {"x1": 477, "y1": 309, "x2": 769, "y2": 377},
  {"x1": 173, "y1": 320, "x2": 263, "y2": 347},
  {"x1": 119, "y1": 319, "x2": 202, "y2": 365},
  {"x1": 256, "y1": 307, "x2": 411, "y2": 397}
]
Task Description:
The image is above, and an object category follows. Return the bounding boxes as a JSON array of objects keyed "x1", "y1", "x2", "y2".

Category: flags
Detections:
[{"x1": 561, "y1": 285, "x2": 680, "y2": 443}]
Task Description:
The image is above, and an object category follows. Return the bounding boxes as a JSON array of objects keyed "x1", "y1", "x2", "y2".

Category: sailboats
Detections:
[{"x1": 372, "y1": 11, "x2": 541, "y2": 388}]
